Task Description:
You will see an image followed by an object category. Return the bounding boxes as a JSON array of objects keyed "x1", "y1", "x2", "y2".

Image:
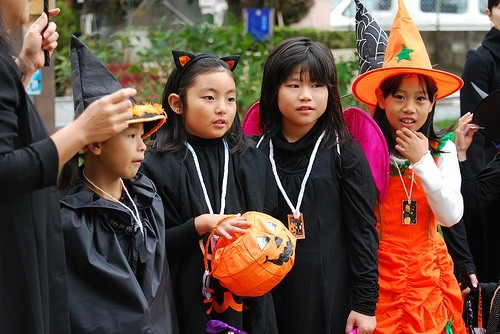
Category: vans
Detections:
[{"x1": 331, "y1": 0, "x2": 494, "y2": 31}]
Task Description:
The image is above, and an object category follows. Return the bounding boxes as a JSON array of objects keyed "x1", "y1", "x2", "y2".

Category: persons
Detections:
[
  {"x1": 437, "y1": 218, "x2": 478, "y2": 297},
  {"x1": 461, "y1": 0, "x2": 500, "y2": 117},
  {"x1": 246, "y1": 37, "x2": 379, "y2": 334},
  {"x1": 430, "y1": 42, "x2": 463, "y2": 76},
  {"x1": 456, "y1": 90, "x2": 500, "y2": 284},
  {"x1": 139, "y1": 51, "x2": 278, "y2": 334},
  {"x1": 0, "y1": 0, "x2": 137, "y2": 334},
  {"x1": 58, "y1": 121, "x2": 178, "y2": 334},
  {"x1": 352, "y1": 74, "x2": 469, "y2": 334}
]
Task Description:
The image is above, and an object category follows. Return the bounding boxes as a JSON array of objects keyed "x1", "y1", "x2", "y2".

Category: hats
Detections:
[
  {"x1": 71, "y1": 35, "x2": 166, "y2": 139},
  {"x1": 355, "y1": 1, "x2": 389, "y2": 75},
  {"x1": 352, "y1": 1, "x2": 463, "y2": 108}
]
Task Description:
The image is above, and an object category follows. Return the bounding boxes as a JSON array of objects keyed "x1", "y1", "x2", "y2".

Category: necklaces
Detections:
[
  {"x1": 269, "y1": 130, "x2": 325, "y2": 239},
  {"x1": 83, "y1": 168, "x2": 144, "y2": 242},
  {"x1": 175, "y1": 134, "x2": 229, "y2": 252},
  {"x1": 392, "y1": 152, "x2": 417, "y2": 224}
]
